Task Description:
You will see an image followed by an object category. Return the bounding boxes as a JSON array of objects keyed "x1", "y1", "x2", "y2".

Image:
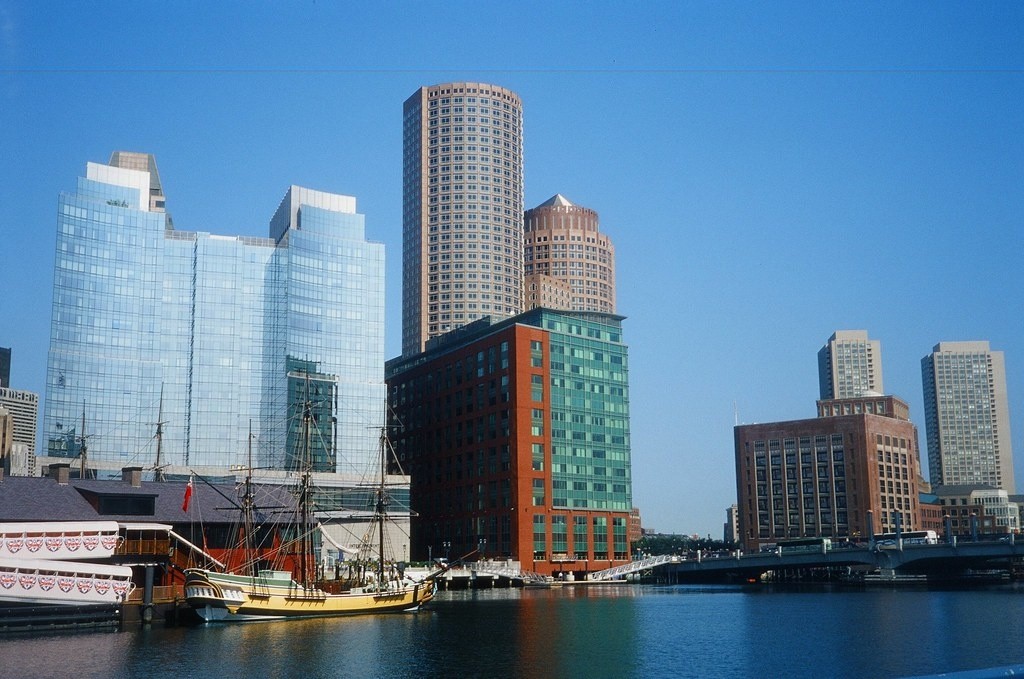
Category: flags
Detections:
[{"x1": 182, "y1": 486, "x2": 192, "y2": 512}]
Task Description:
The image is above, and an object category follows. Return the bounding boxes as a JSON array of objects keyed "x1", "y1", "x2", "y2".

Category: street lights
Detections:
[
  {"x1": 788, "y1": 526, "x2": 792, "y2": 551},
  {"x1": 747, "y1": 530, "x2": 751, "y2": 555}
]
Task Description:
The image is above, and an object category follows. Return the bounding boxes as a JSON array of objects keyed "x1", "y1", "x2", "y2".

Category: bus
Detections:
[
  {"x1": 874, "y1": 530, "x2": 938, "y2": 544},
  {"x1": 775, "y1": 537, "x2": 832, "y2": 552}
]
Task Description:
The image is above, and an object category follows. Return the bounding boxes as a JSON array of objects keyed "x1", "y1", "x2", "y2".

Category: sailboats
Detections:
[{"x1": 183, "y1": 353, "x2": 481, "y2": 622}]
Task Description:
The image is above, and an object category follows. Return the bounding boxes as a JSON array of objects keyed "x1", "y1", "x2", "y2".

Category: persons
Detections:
[{"x1": 398, "y1": 561, "x2": 405, "y2": 580}]
